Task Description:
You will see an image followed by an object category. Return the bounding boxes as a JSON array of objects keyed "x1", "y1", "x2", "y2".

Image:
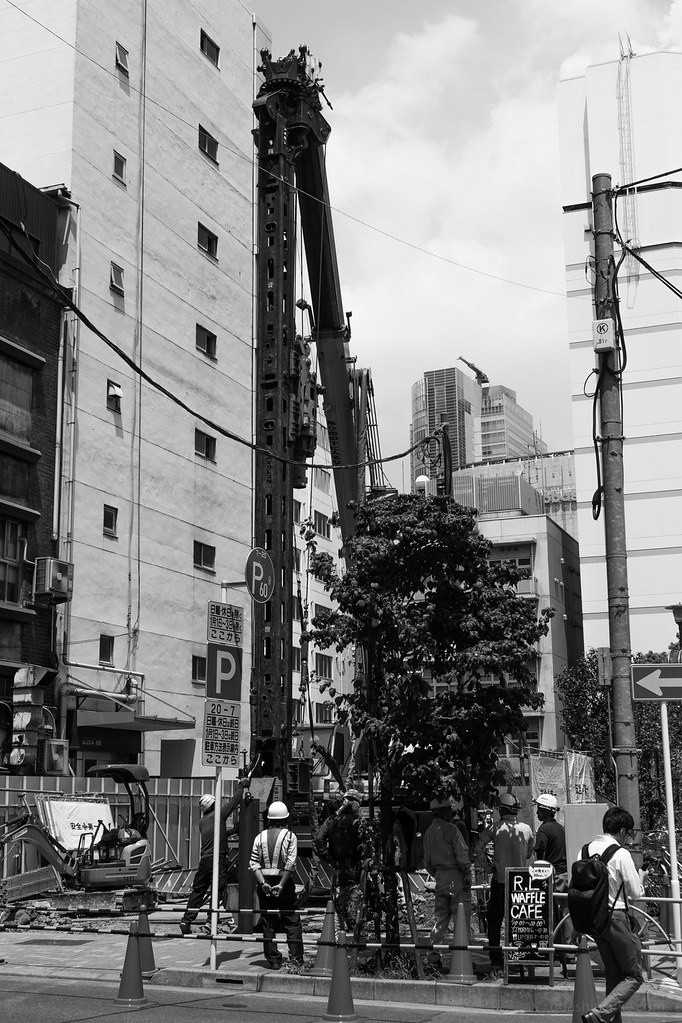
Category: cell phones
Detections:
[{"x1": 641, "y1": 863, "x2": 651, "y2": 872}]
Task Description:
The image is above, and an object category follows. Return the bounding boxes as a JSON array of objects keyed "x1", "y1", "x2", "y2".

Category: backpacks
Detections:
[{"x1": 568, "y1": 842, "x2": 622, "y2": 934}]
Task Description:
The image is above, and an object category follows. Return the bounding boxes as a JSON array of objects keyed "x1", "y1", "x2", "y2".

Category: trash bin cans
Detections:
[{"x1": 227, "y1": 883, "x2": 261, "y2": 926}]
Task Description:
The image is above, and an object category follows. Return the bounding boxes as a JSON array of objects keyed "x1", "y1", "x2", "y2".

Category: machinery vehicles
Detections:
[
  {"x1": 0, "y1": 763, "x2": 162, "y2": 918},
  {"x1": 249, "y1": 40, "x2": 433, "y2": 910}
]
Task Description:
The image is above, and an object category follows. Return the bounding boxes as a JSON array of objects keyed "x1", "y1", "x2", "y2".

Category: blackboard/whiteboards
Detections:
[{"x1": 505, "y1": 867, "x2": 554, "y2": 965}]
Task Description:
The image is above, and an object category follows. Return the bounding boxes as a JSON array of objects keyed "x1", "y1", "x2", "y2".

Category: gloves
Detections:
[{"x1": 240, "y1": 778, "x2": 249, "y2": 788}]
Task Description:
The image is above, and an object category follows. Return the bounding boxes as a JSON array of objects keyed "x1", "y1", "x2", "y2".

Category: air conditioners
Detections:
[
  {"x1": 36, "y1": 739, "x2": 68, "y2": 776},
  {"x1": 34, "y1": 556, "x2": 74, "y2": 604}
]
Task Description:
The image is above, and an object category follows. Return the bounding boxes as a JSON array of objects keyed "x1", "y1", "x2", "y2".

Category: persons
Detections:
[
  {"x1": 180, "y1": 779, "x2": 250, "y2": 933},
  {"x1": 578, "y1": 808, "x2": 649, "y2": 1023},
  {"x1": 249, "y1": 802, "x2": 305, "y2": 969},
  {"x1": 536, "y1": 794, "x2": 573, "y2": 945},
  {"x1": 477, "y1": 793, "x2": 535, "y2": 966},
  {"x1": 316, "y1": 788, "x2": 366, "y2": 931},
  {"x1": 424, "y1": 798, "x2": 476, "y2": 966}
]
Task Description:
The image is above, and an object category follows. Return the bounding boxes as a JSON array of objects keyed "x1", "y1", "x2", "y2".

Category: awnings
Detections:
[{"x1": 109, "y1": 383, "x2": 123, "y2": 399}]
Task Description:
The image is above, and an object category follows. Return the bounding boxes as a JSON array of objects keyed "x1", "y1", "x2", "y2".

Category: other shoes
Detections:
[
  {"x1": 203, "y1": 923, "x2": 211, "y2": 934},
  {"x1": 491, "y1": 959, "x2": 504, "y2": 969},
  {"x1": 268, "y1": 963, "x2": 279, "y2": 970},
  {"x1": 428, "y1": 960, "x2": 442, "y2": 968},
  {"x1": 180, "y1": 923, "x2": 192, "y2": 934}
]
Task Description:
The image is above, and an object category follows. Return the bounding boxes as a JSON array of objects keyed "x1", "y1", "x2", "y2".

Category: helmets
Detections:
[
  {"x1": 267, "y1": 801, "x2": 290, "y2": 819},
  {"x1": 199, "y1": 794, "x2": 215, "y2": 812},
  {"x1": 495, "y1": 793, "x2": 522, "y2": 810},
  {"x1": 430, "y1": 798, "x2": 451, "y2": 809},
  {"x1": 537, "y1": 793, "x2": 557, "y2": 812},
  {"x1": 342, "y1": 789, "x2": 362, "y2": 805}
]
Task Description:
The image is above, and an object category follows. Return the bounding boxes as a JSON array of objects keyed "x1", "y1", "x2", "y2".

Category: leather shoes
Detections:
[{"x1": 582, "y1": 1011, "x2": 601, "y2": 1023}]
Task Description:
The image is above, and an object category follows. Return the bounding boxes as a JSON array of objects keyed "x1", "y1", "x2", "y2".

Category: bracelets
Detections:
[{"x1": 260, "y1": 881, "x2": 267, "y2": 886}]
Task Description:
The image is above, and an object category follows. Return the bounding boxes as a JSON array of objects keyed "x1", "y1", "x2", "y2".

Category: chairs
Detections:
[{"x1": 104, "y1": 811, "x2": 146, "y2": 841}]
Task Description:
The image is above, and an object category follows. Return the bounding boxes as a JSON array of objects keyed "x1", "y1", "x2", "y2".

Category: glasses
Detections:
[{"x1": 625, "y1": 830, "x2": 632, "y2": 839}]
